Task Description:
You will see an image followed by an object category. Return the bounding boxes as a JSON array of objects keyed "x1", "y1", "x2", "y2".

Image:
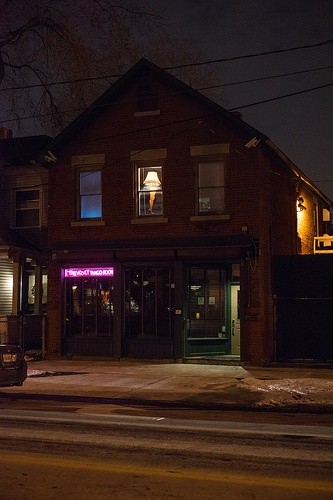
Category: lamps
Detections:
[{"x1": 143, "y1": 168, "x2": 163, "y2": 214}]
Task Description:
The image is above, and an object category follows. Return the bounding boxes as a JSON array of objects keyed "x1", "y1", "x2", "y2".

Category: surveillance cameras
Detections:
[
  {"x1": 298, "y1": 204, "x2": 307, "y2": 210},
  {"x1": 245, "y1": 137, "x2": 258, "y2": 149},
  {"x1": 41, "y1": 150, "x2": 59, "y2": 165}
]
